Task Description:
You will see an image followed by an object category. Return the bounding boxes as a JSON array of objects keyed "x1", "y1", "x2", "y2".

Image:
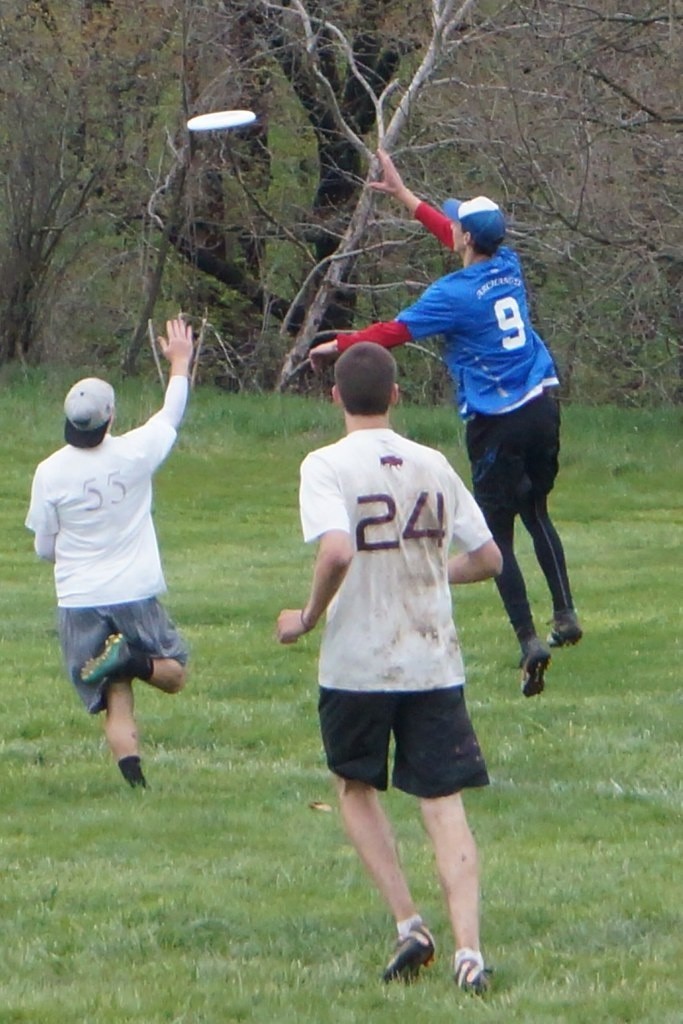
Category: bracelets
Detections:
[{"x1": 300, "y1": 609, "x2": 315, "y2": 629}]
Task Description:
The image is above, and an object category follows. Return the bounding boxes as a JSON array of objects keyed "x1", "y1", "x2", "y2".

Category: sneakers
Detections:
[
  {"x1": 81, "y1": 632, "x2": 131, "y2": 685},
  {"x1": 454, "y1": 956, "x2": 492, "y2": 997},
  {"x1": 520, "y1": 647, "x2": 552, "y2": 697},
  {"x1": 382, "y1": 921, "x2": 435, "y2": 984},
  {"x1": 546, "y1": 609, "x2": 583, "y2": 648}
]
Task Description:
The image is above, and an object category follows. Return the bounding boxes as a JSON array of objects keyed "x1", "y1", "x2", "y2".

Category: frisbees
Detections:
[{"x1": 187, "y1": 110, "x2": 256, "y2": 130}]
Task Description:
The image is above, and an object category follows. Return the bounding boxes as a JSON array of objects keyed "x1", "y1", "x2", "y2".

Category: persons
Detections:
[
  {"x1": 305, "y1": 147, "x2": 582, "y2": 696},
  {"x1": 278, "y1": 340, "x2": 506, "y2": 1001},
  {"x1": 26, "y1": 316, "x2": 194, "y2": 791}
]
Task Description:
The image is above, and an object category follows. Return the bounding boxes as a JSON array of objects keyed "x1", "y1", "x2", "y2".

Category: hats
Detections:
[
  {"x1": 443, "y1": 196, "x2": 506, "y2": 246},
  {"x1": 63, "y1": 376, "x2": 116, "y2": 449}
]
